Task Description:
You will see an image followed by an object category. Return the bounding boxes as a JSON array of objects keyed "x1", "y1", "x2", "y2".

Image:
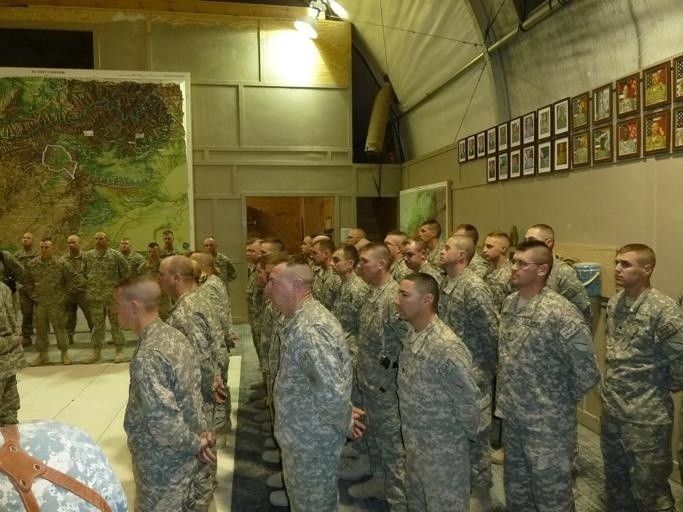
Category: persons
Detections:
[
  {"x1": 525, "y1": 118, "x2": 533, "y2": 138},
  {"x1": 646, "y1": 122, "x2": 667, "y2": 151},
  {"x1": 479, "y1": 232, "x2": 520, "y2": 313},
  {"x1": 346, "y1": 228, "x2": 366, "y2": 245},
  {"x1": 499, "y1": 127, "x2": 506, "y2": 145},
  {"x1": 137, "y1": 242, "x2": 173, "y2": 322},
  {"x1": 512, "y1": 157, "x2": 519, "y2": 174},
  {"x1": 244, "y1": 237, "x2": 285, "y2": 462},
  {"x1": 393, "y1": 272, "x2": 483, "y2": 512},
  {"x1": 595, "y1": 244, "x2": 683, "y2": 512},
  {"x1": 557, "y1": 142, "x2": 567, "y2": 166},
  {"x1": 460, "y1": 143, "x2": 465, "y2": 159},
  {"x1": 454, "y1": 224, "x2": 489, "y2": 279},
  {"x1": 598, "y1": 127, "x2": 609, "y2": 142},
  {"x1": 618, "y1": 126, "x2": 638, "y2": 156},
  {"x1": 114, "y1": 274, "x2": 217, "y2": 512},
  {"x1": 573, "y1": 102, "x2": 587, "y2": 128},
  {"x1": 494, "y1": 239, "x2": 602, "y2": 512},
  {"x1": 478, "y1": 136, "x2": 484, "y2": 153},
  {"x1": 500, "y1": 160, "x2": 506, "y2": 175},
  {"x1": 541, "y1": 148, "x2": 549, "y2": 168},
  {"x1": 347, "y1": 241, "x2": 415, "y2": 512},
  {"x1": 267, "y1": 255, "x2": 367, "y2": 512},
  {"x1": 433, "y1": 235, "x2": 499, "y2": 512},
  {"x1": 540, "y1": 113, "x2": 549, "y2": 136},
  {"x1": 400, "y1": 236, "x2": 437, "y2": 274},
  {"x1": 0, "y1": 250, "x2": 24, "y2": 331},
  {"x1": 489, "y1": 162, "x2": 495, "y2": 178},
  {"x1": 0, "y1": 419, "x2": 129, "y2": 512},
  {"x1": 310, "y1": 239, "x2": 341, "y2": 310},
  {"x1": 24, "y1": 237, "x2": 77, "y2": 366},
  {"x1": 512, "y1": 123, "x2": 519, "y2": 143},
  {"x1": 645, "y1": 71, "x2": 667, "y2": 106},
  {"x1": 158, "y1": 231, "x2": 181, "y2": 258},
  {"x1": 80, "y1": 232, "x2": 130, "y2": 363},
  {"x1": 119, "y1": 238, "x2": 146, "y2": 276},
  {"x1": 619, "y1": 84, "x2": 637, "y2": 114},
  {"x1": 575, "y1": 137, "x2": 587, "y2": 164},
  {"x1": 61, "y1": 234, "x2": 92, "y2": 345},
  {"x1": 489, "y1": 133, "x2": 495, "y2": 150},
  {"x1": 468, "y1": 141, "x2": 475, "y2": 157},
  {"x1": 189, "y1": 250, "x2": 239, "y2": 354},
  {"x1": 191, "y1": 259, "x2": 232, "y2": 449},
  {"x1": 330, "y1": 243, "x2": 373, "y2": 480},
  {"x1": 12, "y1": 233, "x2": 40, "y2": 348},
  {"x1": 160, "y1": 255, "x2": 228, "y2": 512},
  {"x1": 599, "y1": 96, "x2": 610, "y2": 120},
  {"x1": 557, "y1": 105, "x2": 567, "y2": 130},
  {"x1": 524, "y1": 224, "x2": 593, "y2": 333},
  {"x1": 0, "y1": 280, "x2": 25, "y2": 427},
  {"x1": 595, "y1": 137, "x2": 610, "y2": 160},
  {"x1": 419, "y1": 219, "x2": 445, "y2": 274},
  {"x1": 525, "y1": 149, "x2": 534, "y2": 169},
  {"x1": 203, "y1": 238, "x2": 240, "y2": 339},
  {"x1": 302, "y1": 233, "x2": 316, "y2": 265},
  {"x1": 384, "y1": 231, "x2": 412, "y2": 280}
]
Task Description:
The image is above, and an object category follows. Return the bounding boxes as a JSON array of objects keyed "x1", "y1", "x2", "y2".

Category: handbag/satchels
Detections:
[{"x1": 0, "y1": 253, "x2": 17, "y2": 292}]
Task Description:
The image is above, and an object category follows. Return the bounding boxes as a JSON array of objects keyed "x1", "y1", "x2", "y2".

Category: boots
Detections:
[
  {"x1": 265, "y1": 437, "x2": 277, "y2": 447},
  {"x1": 29, "y1": 352, "x2": 49, "y2": 366},
  {"x1": 255, "y1": 398, "x2": 269, "y2": 408},
  {"x1": 263, "y1": 421, "x2": 274, "y2": 431},
  {"x1": 341, "y1": 443, "x2": 357, "y2": 457},
  {"x1": 348, "y1": 478, "x2": 385, "y2": 498},
  {"x1": 491, "y1": 447, "x2": 505, "y2": 463},
  {"x1": 83, "y1": 349, "x2": 103, "y2": 363},
  {"x1": 62, "y1": 350, "x2": 72, "y2": 364},
  {"x1": 255, "y1": 410, "x2": 272, "y2": 421},
  {"x1": 262, "y1": 450, "x2": 282, "y2": 463},
  {"x1": 20, "y1": 336, "x2": 31, "y2": 345},
  {"x1": 250, "y1": 389, "x2": 265, "y2": 399},
  {"x1": 340, "y1": 455, "x2": 371, "y2": 477},
  {"x1": 270, "y1": 490, "x2": 291, "y2": 506},
  {"x1": 469, "y1": 490, "x2": 491, "y2": 511},
  {"x1": 114, "y1": 352, "x2": 124, "y2": 362},
  {"x1": 268, "y1": 472, "x2": 287, "y2": 487},
  {"x1": 251, "y1": 381, "x2": 265, "y2": 389}
]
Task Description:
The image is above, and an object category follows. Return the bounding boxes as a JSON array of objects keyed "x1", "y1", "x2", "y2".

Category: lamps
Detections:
[{"x1": 294, "y1": 0, "x2": 327, "y2": 39}]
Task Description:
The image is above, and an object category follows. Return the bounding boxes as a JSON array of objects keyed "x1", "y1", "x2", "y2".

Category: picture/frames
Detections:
[
  {"x1": 643, "y1": 109, "x2": 670, "y2": 156},
  {"x1": 673, "y1": 55, "x2": 683, "y2": 102},
  {"x1": 536, "y1": 141, "x2": 552, "y2": 175},
  {"x1": 592, "y1": 125, "x2": 614, "y2": 164},
  {"x1": 552, "y1": 135, "x2": 570, "y2": 172},
  {"x1": 521, "y1": 145, "x2": 537, "y2": 177},
  {"x1": 570, "y1": 90, "x2": 590, "y2": 130},
  {"x1": 535, "y1": 104, "x2": 552, "y2": 143},
  {"x1": 571, "y1": 131, "x2": 590, "y2": 168},
  {"x1": 592, "y1": 83, "x2": 613, "y2": 124},
  {"x1": 551, "y1": 97, "x2": 570, "y2": 139},
  {"x1": 616, "y1": 72, "x2": 640, "y2": 119},
  {"x1": 509, "y1": 149, "x2": 522, "y2": 178},
  {"x1": 476, "y1": 130, "x2": 487, "y2": 157},
  {"x1": 396, "y1": 181, "x2": 452, "y2": 246},
  {"x1": 497, "y1": 152, "x2": 509, "y2": 181},
  {"x1": 458, "y1": 138, "x2": 467, "y2": 163},
  {"x1": 497, "y1": 121, "x2": 509, "y2": 154},
  {"x1": 616, "y1": 117, "x2": 641, "y2": 160},
  {"x1": 467, "y1": 134, "x2": 476, "y2": 160},
  {"x1": 509, "y1": 116, "x2": 522, "y2": 150},
  {"x1": 487, "y1": 155, "x2": 497, "y2": 183},
  {"x1": 0, "y1": 61, "x2": 197, "y2": 332},
  {"x1": 521, "y1": 111, "x2": 536, "y2": 146},
  {"x1": 643, "y1": 61, "x2": 671, "y2": 110},
  {"x1": 672, "y1": 107, "x2": 683, "y2": 151},
  {"x1": 486, "y1": 126, "x2": 498, "y2": 156}
]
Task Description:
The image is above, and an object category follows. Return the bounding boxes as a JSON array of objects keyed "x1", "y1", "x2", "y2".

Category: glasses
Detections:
[
  {"x1": 511, "y1": 260, "x2": 547, "y2": 267},
  {"x1": 346, "y1": 234, "x2": 361, "y2": 239}
]
